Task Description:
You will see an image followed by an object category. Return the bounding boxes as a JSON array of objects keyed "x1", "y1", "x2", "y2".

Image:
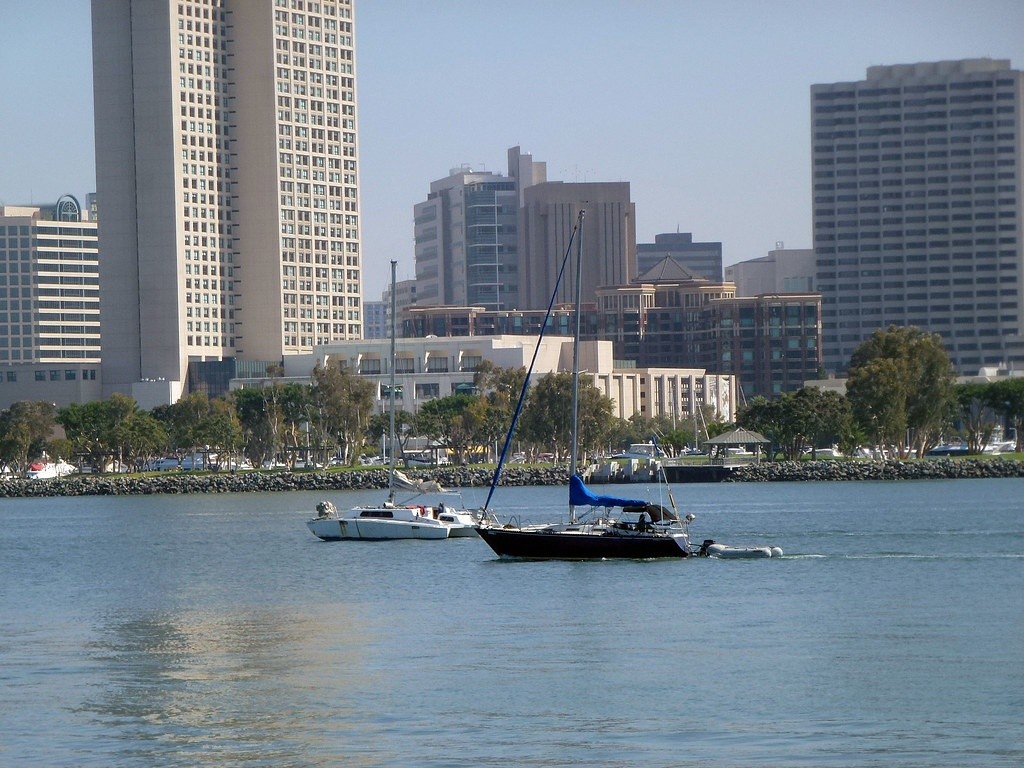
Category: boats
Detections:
[{"x1": 706, "y1": 543, "x2": 785, "y2": 559}]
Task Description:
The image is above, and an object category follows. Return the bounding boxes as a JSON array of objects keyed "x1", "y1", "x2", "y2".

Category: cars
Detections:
[{"x1": 926, "y1": 443, "x2": 971, "y2": 457}]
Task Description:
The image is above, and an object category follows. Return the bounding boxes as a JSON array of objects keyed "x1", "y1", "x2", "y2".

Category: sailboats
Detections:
[
  {"x1": 467, "y1": 207, "x2": 697, "y2": 561},
  {"x1": 304, "y1": 257, "x2": 494, "y2": 541}
]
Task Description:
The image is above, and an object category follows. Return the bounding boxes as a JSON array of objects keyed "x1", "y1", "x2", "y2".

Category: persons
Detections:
[
  {"x1": 590, "y1": 453, "x2": 608, "y2": 465},
  {"x1": 437, "y1": 502, "x2": 444, "y2": 516},
  {"x1": 389, "y1": 490, "x2": 397, "y2": 505},
  {"x1": 636, "y1": 514, "x2": 653, "y2": 531}
]
